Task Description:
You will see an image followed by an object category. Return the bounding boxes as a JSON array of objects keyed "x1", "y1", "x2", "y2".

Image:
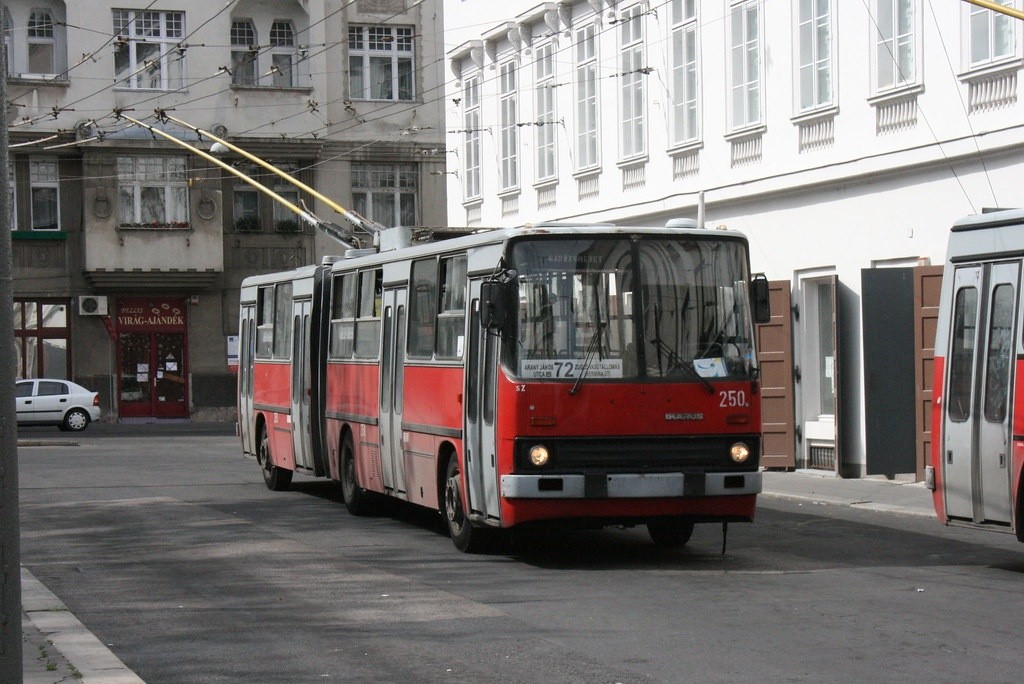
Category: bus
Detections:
[
  {"x1": 926, "y1": 208, "x2": 1023, "y2": 545},
  {"x1": 236, "y1": 215, "x2": 771, "y2": 552}
]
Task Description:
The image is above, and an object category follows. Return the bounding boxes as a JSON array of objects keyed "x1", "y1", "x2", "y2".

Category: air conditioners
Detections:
[{"x1": 78, "y1": 295, "x2": 108, "y2": 316}]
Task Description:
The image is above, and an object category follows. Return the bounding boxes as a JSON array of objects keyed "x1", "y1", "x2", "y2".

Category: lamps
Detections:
[{"x1": 209, "y1": 142, "x2": 230, "y2": 154}]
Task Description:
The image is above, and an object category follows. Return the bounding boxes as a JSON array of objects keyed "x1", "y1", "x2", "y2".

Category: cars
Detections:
[{"x1": 16, "y1": 379, "x2": 101, "y2": 432}]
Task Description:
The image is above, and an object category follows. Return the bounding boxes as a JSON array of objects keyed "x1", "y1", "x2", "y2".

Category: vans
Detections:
[{"x1": 120, "y1": 377, "x2": 147, "y2": 403}]
